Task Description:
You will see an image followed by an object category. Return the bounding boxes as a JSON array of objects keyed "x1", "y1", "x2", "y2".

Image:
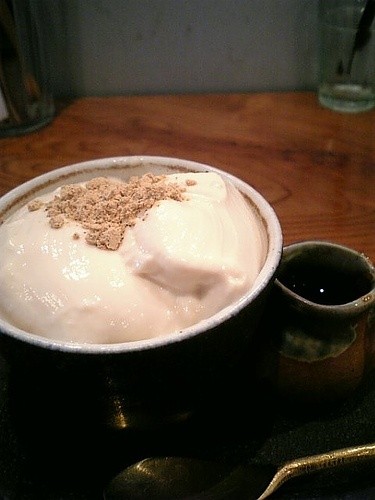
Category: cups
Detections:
[
  {"x1": 318, "y1": 0, "x2": 374, "y2": 112},
  {"x1": 0, "y1": 0, "x2": 53, "y2": 137},
  {"x1": 269, "y1": 242, "x2": 374, "y2": 394}
]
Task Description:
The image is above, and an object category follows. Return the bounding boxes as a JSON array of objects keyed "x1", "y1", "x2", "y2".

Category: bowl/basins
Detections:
[{"x1": 0, "y1": 156, "x2": 283, "y2": 353}]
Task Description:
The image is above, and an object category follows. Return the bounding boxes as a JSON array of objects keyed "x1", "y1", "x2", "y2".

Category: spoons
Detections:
[{"x1": 101, "y1": 444, "x2": 375, "y2": 500}]
